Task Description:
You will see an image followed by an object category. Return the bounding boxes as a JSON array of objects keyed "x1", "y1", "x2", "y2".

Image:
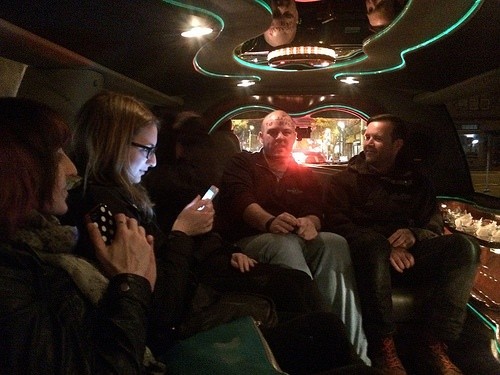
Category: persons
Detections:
[
  {"x1": 143, "y1": 109, "x2": 312, "y2": 314},
  {"x1": 322, "y1": 114, "x2": 481, "y2": 375},
  {"x1": 224, "y1": 110, "x2": 372, "y2": 375},
  {"x1": 263, "y1": 0, "x2": 299, "y2": 47},
  {"x1": 366, "y1": 0, "x2": 402, "y2": 34},
  {"x1": 57, "y1": 87, "x2": 374, "y2": 375},
  {"x1": 0, "y1": 96, "x2": 167, "y2": 375}
]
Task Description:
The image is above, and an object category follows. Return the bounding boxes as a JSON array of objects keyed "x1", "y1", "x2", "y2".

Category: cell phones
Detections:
[
  {"x1": 86, "y1": 203, "x2": 117, "y2": 246},
  {"x1": 197, "y1": 185, "x2": 219, "y2": 211}
]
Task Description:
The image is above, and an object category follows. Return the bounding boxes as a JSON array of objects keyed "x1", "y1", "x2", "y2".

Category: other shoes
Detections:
[
  {"x1": 425, "y1": 337, "x2": 463, "y2": 375},
  {"x1": 378, "y1": 337, "x2": 408, "y2": 375}
]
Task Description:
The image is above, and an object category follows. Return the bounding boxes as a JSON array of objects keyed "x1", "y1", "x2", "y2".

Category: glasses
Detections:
[{"x1": 130, "y1": 141, "x2": 159, "y2": 159}]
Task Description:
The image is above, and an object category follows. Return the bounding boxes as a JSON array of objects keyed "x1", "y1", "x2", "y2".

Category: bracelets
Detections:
[{"x1": 265, "y1": 218, "x2": 276, "y2": 233}]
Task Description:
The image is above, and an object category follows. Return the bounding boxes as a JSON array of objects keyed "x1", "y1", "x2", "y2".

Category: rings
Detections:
[{"x1": 117, "y1": 220, "x2": 128, "y2": 225}]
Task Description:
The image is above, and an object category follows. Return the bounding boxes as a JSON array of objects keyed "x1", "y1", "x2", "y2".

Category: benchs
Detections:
[{"x1": 308, "y1": 166, "x2": 419, "y2": 321}]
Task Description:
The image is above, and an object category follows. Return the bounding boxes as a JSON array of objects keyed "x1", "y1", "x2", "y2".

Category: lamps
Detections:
[{"x1": 267, "y1": 43, "x2": 337, "y2": 70}]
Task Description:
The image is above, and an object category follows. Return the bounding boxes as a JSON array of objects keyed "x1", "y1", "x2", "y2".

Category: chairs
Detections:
[{"x1": 212, "y1": 129, "x2": 243, "y2": 166}]
